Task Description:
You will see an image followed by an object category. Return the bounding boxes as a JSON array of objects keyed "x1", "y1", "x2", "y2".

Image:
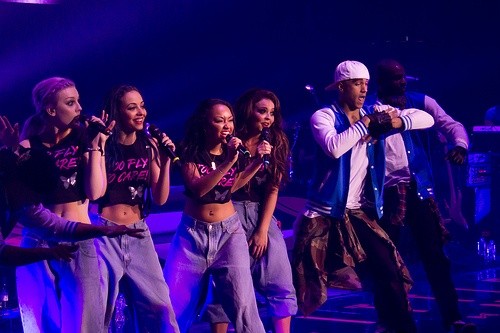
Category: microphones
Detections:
[
  {"x1": 261, "y1": 127, "x2": 270, "y2": 165},
  {"x1": 148, "y1": 124, "x2": 180, "y2": 165},
  {"x1": 226, "y1": 134, "x2": 251, "y2": 158},
  {"x1": 78, "y1": 115, "x2": 112, "y2": 136}
]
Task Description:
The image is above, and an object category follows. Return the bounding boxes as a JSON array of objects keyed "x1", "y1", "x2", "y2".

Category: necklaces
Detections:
[{"x1": 202, "y1": 146, "x2": 223, "y2": 171}]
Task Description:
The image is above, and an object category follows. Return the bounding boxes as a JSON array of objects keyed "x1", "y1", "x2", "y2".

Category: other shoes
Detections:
[{"x1": 446, "y1": 320, "x2": 475, "y2": 333}]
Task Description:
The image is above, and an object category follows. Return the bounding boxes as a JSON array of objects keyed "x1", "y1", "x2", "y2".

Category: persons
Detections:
[
  {"x1": 163, "y1": 98, "x2": 266, "y2": 333},
  {"x1": 371, "y1": 61, "x2": 476, "y2": 333},
  {"x1": 291, "y1": 60, "x2": 435, "y2": 333},
  {"x1": 98, "y1": 85, "x2": 180, "y2": 333},
  {"x1": 0, "y1": 202, "x2": 144, "y2": 266},
  {"x1": 203, "y1": 89, "x2": 298, "y2": 333},
  {"x1": 0, "y1": 77, "x2": 106, "y2": 333}
]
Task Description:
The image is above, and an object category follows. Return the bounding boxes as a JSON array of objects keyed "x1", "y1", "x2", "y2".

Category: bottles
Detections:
[
  {"x1": 476, "y1": 237, "x2": 487, "y2": 280},
  {"x1": 487, "y1": 239, "x2": 497, "y2": 280}
]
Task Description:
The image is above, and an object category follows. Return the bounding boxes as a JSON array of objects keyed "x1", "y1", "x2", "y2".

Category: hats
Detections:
[{"x1": 325, "y1": 61, "x2": 370, "y2": 90}]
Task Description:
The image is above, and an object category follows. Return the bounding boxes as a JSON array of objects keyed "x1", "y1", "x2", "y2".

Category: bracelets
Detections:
[
  {"x1": 215, "y1": 167, "x2": 225, "y2": 177},
  {"x1": 87, "y1": 146, "x2": 101, "y2": 151}
]
